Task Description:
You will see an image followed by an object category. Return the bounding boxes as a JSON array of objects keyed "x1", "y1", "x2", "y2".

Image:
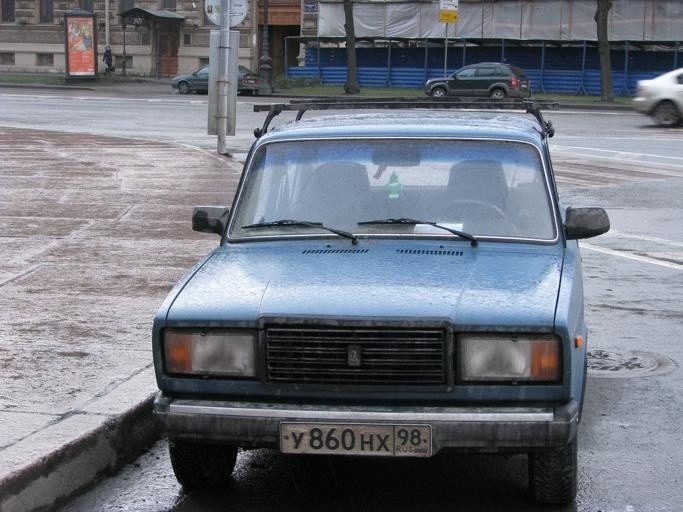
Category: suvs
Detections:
[{"x1": 419, "y1": 60, "x2": 533, "y2": 106}]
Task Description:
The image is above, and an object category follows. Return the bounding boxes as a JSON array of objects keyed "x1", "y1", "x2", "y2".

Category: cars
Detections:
[
  {"x1": 150, "y1": 99, "x2": 613, "y2": 497},
  {"x1": 169, "y1": 60, "x2": 263, "y2": 95},
  {"x1": 630, "y1": 66, "x2": 682, "y2": 126}
]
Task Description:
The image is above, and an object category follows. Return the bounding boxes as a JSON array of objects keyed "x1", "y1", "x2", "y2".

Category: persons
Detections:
[{"x1": 101, "y1": 45, "x2": 115, "y2": 80}]
